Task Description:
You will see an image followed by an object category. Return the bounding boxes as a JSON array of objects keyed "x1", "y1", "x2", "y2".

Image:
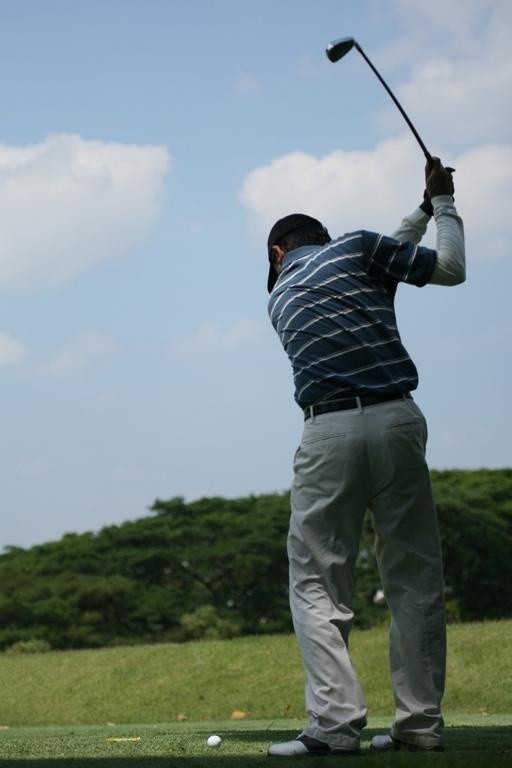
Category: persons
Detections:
[{"x1": 263, "y1": 153, "x2": 471, "y2": 756}]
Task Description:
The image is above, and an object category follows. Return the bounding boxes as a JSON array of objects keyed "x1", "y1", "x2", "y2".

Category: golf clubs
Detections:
[{"x1": 326, "y1": 36, "x2": 433, "y2": 163}]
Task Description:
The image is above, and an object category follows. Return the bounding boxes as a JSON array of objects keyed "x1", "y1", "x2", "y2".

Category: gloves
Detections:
[{"x1": 418, "y1": 156, "x2": 457, "y2": 215}]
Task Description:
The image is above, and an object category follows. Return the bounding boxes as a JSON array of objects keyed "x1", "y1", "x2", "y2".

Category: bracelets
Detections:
[{"x1": 419, "y1": 197, "x2": 435, "y2": 217}]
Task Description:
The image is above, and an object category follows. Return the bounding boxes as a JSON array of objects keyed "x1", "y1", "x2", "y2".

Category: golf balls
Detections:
[
  {"x1": 207, "y1": 735, "x2": 221, "y2": 748},
  {"x1": 370, "y1": 733, "x2": 388, "y2": 750}
]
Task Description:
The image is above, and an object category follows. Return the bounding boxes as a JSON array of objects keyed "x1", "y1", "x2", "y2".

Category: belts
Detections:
[{"x1": 304, "y1": 390, "x2": 412, "y2": 421}]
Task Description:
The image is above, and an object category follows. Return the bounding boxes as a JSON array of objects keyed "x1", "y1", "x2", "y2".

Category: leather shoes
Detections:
[
  {"x1": 266, "y1": 733, "x2": 360, "y2": 757},
  {"x1": 371, "y1": 730, "x2": 444, "y2": 751}
]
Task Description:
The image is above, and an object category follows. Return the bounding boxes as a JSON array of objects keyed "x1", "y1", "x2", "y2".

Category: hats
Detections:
[{"x1": 265, "y1": 213, "x2": 326, "y2": 294}]
